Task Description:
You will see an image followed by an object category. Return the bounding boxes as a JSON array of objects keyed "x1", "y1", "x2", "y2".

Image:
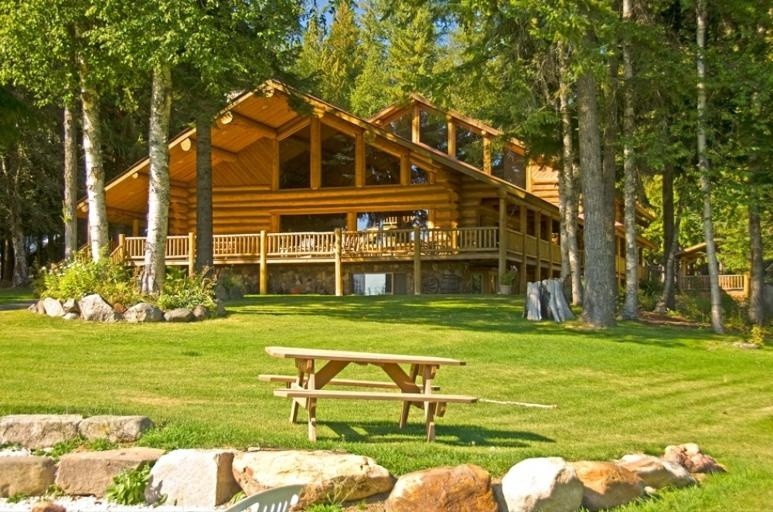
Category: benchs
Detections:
[
  {"x1": 257, "y1": 373, "x2": 443, "y2": 430},
  {"x1": 274, "y1": 385, "x2": 478, "y2": 443}
]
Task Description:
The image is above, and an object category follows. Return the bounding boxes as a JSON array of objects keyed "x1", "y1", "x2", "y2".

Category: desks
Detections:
[{"x1": 264, "y1": 344, "x2": 467, "y2": 446}]
